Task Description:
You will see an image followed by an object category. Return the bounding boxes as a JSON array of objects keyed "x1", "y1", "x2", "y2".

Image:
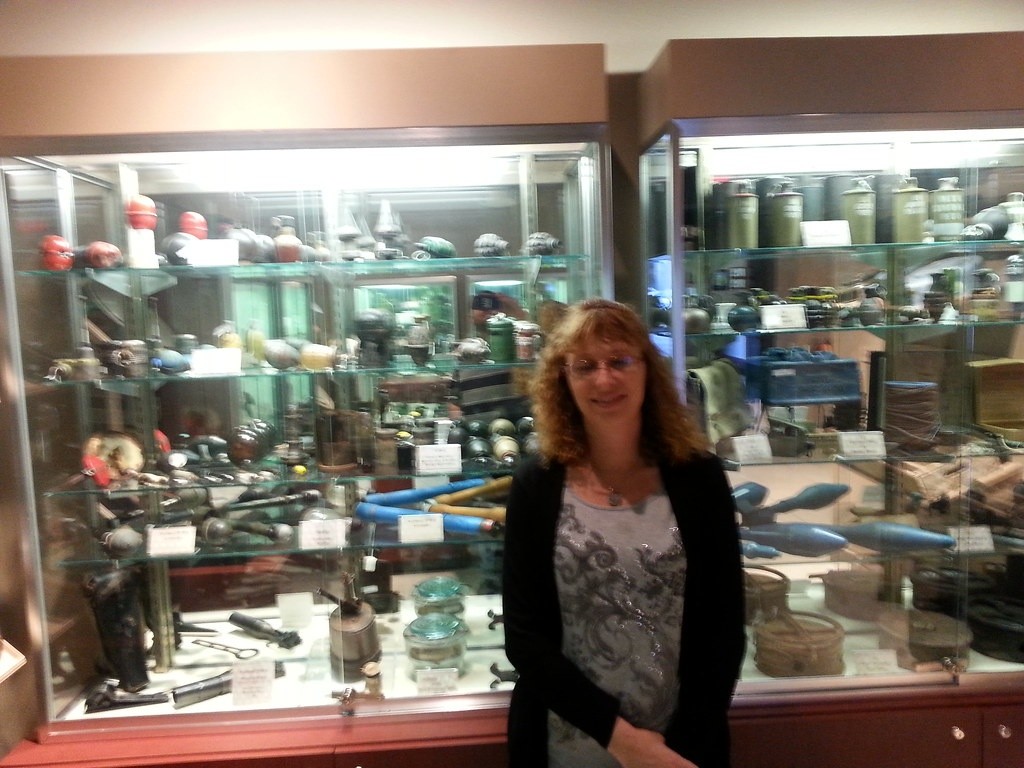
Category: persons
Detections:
[
  {"x1": 502, "y1": 297, "x2": 748, "y2": 768},
  {"x1": 445, "y1": 291, "x2": 571, "y2": 427}
]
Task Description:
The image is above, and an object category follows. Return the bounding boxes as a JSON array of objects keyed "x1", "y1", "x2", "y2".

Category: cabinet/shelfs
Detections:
[
  {"x1": 636, "y1": 30, "x2": 1024, "y2": 768},
  {"x1": 0, "y1": 42, "x2": 619, "y2": 768}
]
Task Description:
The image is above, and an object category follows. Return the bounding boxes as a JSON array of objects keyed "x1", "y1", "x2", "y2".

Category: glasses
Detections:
[{"x1": 563, "y1": 353, "x2": 634, "y2": 374}]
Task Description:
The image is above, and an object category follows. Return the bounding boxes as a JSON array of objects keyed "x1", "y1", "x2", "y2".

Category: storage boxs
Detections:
[{"x1": 747, "y1": 355, "x2": 862, "y2": 403}]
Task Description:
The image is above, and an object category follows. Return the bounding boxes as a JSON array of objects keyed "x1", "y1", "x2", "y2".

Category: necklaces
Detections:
[{"x1": 589, "y1": 458, "x2": 646, "y2": 507}]
